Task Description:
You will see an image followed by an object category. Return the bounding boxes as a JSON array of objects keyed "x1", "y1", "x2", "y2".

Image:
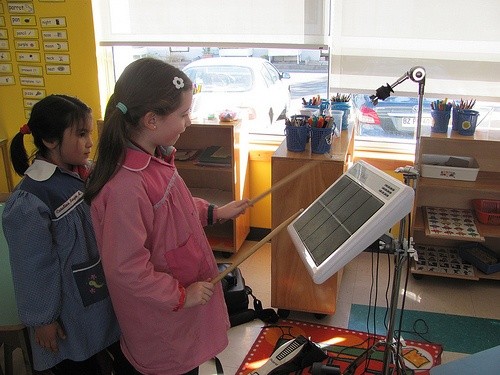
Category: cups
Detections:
[
  {"x1": 430, "y1": 107, "x2": 479, "y2": 136},
  {"x1": 300, "y1": 98, "x2": 352, "y2": 139}
]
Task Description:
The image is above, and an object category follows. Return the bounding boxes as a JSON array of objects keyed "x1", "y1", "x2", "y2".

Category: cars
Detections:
[
  {"x1": 180, "y1": 55, "x2": 293, "y2": 128},
  {"x1": 349, "y1": 94, "x2": 432, "y2": 140}
]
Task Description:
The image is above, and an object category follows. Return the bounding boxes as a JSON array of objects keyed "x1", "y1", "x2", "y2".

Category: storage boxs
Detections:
[{"x1": 419, "y1": 153, "x2": 480, "y2": 181}]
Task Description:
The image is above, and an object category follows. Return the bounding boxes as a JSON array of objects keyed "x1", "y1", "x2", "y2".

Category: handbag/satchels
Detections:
[{"x1": 218, "y1": 264, "x2": 279, "y2": 327}]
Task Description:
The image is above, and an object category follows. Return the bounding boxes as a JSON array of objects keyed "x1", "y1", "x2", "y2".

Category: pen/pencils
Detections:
[
  {"x1": 285, "y1": 113, "x2": 335, "y2": 128},
  {"x1": 430, "y1": 97, "x2": 476, "y2": 110},
  {"x1": 192, "y1": 82, "x2": 202, "y2": 95},
  {"x1": 302, "y1": 95, "x2": 321, "y2": 105},
  {"x1": 331, "y1": 92, "x2": 352, "y2": 102}
]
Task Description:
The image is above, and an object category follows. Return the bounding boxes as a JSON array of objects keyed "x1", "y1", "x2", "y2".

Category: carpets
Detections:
[
  {"x1": 233, "y1": 318, "x2": 443, "y2": 375},
  {"x1": 346, "y1": 303, "x2": 500, "y2": 355}
]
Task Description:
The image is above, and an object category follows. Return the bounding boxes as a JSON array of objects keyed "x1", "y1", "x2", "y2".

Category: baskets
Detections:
[
  {"x1": 285, "y1": 126, "x2": 307, "y2": 151},
  {"x1": 453, "y1": 109, "x2": 458, "y2": 130},
  {"x1": 311, "y1": 127, "x2": 331, "y2": 153},
  {"x1": 473, "y1": 200, "x2": 500, "y2": 225}
]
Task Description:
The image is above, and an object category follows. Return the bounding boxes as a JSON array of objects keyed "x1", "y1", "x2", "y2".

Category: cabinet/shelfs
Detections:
[
  {"x1": 268, "y1": 123, "x2": 356, "y2": 314},
  {"x1": 98, "y1": 111, "x2": 252, "y2": 254},
  {"x1": 408, "y1": 126, "x2": 500, "y2": 283}
]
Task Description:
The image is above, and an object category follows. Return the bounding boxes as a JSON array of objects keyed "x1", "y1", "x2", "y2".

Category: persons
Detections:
[
  {"x1": 84, "y1": 57, "x2": 255, "y2": 375},
  {"x1": 2, "y1": 94, "x2": 123, "y2": 375}
]
